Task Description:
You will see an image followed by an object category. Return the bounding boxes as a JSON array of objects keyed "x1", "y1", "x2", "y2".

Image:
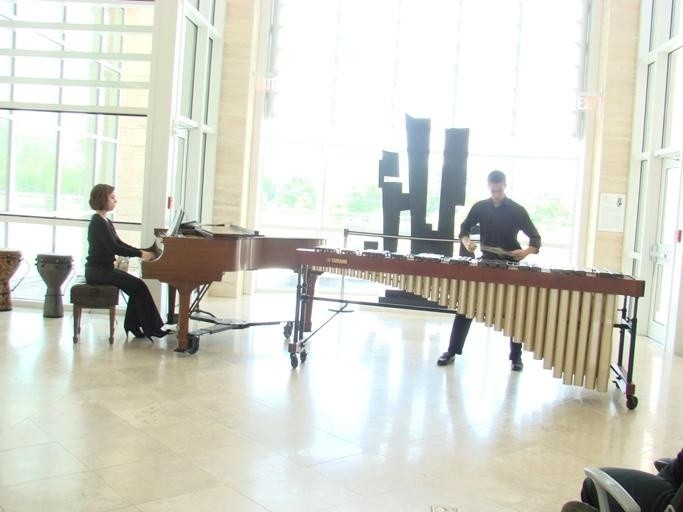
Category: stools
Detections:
[{"x1": 67, "y1": 282, "x2": 117, "y2": 348}]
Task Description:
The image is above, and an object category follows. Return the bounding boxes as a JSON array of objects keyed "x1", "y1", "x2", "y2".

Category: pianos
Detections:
[{"x1": 141, "y1": 223, "x2": 326, "y2": 351}]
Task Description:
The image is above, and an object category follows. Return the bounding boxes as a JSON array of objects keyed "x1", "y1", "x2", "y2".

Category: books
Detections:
[{"x1": 168, "y1": 207, "x2": 184, "y2": 237}]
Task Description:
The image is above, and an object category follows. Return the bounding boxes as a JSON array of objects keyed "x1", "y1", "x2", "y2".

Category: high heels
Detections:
[
  {"x1": 124, "y1": 323, "x2": 144, "y2": 338},
  {"x1": 142, "y1": 327, "x2": 170, "y2": 343}
]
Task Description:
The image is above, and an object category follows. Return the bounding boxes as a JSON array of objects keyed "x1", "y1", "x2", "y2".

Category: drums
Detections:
[
  {"x1": 1, "y1": 251, "x2": 21, "y2": 310},
  {"x1": 35, "y1": 255, "x2": 75, "y2": 318}
]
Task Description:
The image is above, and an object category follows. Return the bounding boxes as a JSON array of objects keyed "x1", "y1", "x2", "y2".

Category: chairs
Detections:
[{"x1": 557, "y1": 456, "x2": 683, "y2": 512}]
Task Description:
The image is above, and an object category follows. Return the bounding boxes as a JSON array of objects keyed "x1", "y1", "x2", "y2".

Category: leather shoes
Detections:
[
  {"x1": 437, "y1": 351, "x2": 455, "y2": 366},
  {"x1": 511, "y1": 358, "x2": 523, "y2": 371}
]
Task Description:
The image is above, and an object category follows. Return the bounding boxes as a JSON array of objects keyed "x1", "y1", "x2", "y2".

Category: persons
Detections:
[
  {"x1": 84, "y1": 184, "x2": 169, "y2": 345},
  {"x1": 436, "y1": 169, "x2": 541, "y2": 371}
]
